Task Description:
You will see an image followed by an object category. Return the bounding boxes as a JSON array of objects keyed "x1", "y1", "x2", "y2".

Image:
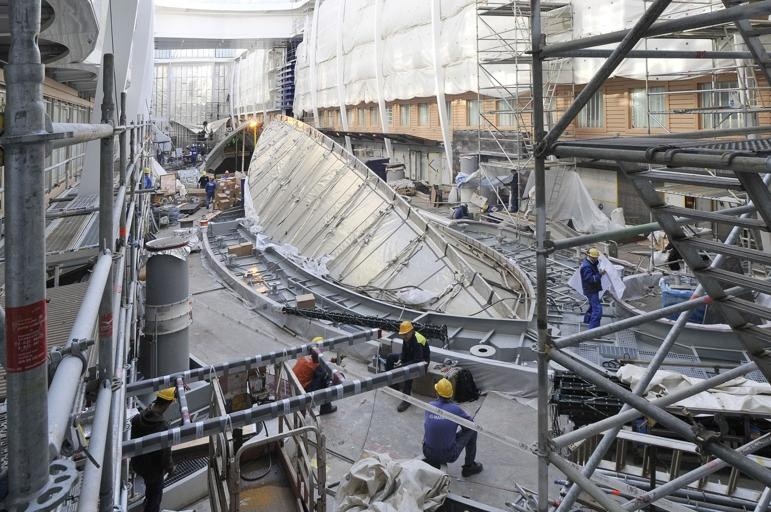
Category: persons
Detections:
[
  {"x1": 503, "y1": 169, "x2": 519, "y2": 213},
  {"x1": 579, "y1": 247, "x2": 607, "y2": 329},
  {"x1": 660, "y1": 241, "x2": 682, "y2": 271},
  {"x1": 287, "y1": 336, "x2": 339, "y2": 416},
  {"x1": 482, "y1": 204, "x2": 503, "y2": 224},
  {"x1": 185, "y1": 142, "x2": 206, "y2": 167},
  {"x1": 380, "y1": 319, "x2": 432, "y2": 413},
  {"x1": 130, "y1": 386, "x2": 178, "y2": 512},
  {"x1": 197, "y1": 170, "x2": 209, "y2": 189},
  {"x1": 421, "y1": 377, "x2": 483, "y2": 478},
  {"x1": 630, "y1": 414, "x2": 661, "y2": 468},
  {"x1": 144, "y1": 166, "x2": 152, "y2": 189},
  {"x1": 204, "y1": 175, "x2": 217, "y2": 210},
  {"x1": 451, "y1": 204, "x2": 468, "y2": 219},
  {"x1": 221, "y1": 169, "x2": 231, "y2": 180}
]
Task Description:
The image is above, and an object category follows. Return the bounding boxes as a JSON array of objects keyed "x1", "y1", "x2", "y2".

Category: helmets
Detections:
[
  {"x1": 460, "y1": 203, "x2": 467, "y2": 208},
  {"x1": 225, "y1": 171, "x2": 229, "y2": 174},
  {"x1": 313, "y1": 337, "x2": 323, "y2": 342},
  {"x1": 144, "y1": 167, "x2": 150, "y2": 174},
  {"x1": 157, "y1": 386, "x2": 176, "y2": 400},
  {"x1": 435, "y1": 377, "x2": 454, "y2": 398},
  {"x1": 588, "y1": 248, "x2": 601, "y2": 258},
  {"x1": 399, "y1": 321, "x2": 414, "y2": 335},
  {"x1": 202, "y1": 171, "x2": 206, "y2": 176},
  {"x1": 210, "y1": 176, "x2": 215, "y2": 179}
]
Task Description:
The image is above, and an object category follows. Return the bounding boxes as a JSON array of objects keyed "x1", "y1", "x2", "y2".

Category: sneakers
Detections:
[
  {"x1": 320, "y1": 406, "x2": 337, "y2": 414},
  {"x1": 462, "y1": 462, "x2": 482, "y2": 477},
  {"x1": 398, "y1": 401, "x2": 410, "y2": 411}
]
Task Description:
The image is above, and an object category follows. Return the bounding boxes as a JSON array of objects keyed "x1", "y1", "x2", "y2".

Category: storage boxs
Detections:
[
  {"x1": 187, "y1": 177, "x2": 241, "y2": 211},
  {"x1": 296, "y1": 293, "x2": 315, "y2": 308},
  {"x1": 228, "y1": 241, "x2": 254, "y2": 258}
]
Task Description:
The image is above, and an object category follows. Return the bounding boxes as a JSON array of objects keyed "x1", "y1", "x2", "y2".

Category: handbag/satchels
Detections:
[{"x1": 455, "y1": 368, "x2": 479, "y2": 402}]
